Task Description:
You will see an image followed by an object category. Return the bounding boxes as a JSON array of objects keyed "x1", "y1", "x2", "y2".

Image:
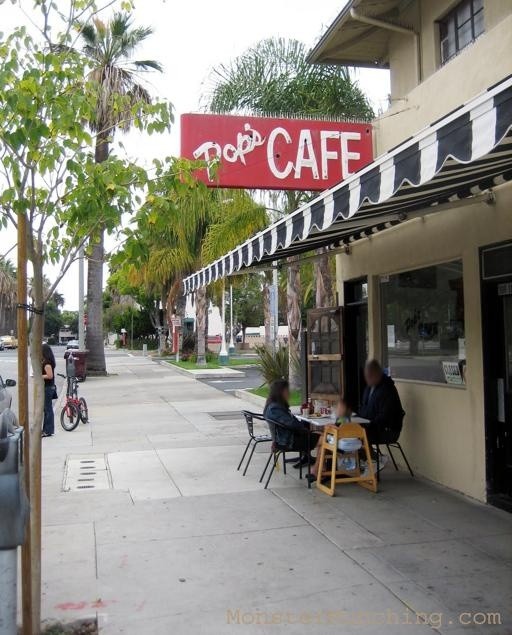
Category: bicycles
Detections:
[{"x1": 57, "y1": 373, "x2": 89, "y2": 430}]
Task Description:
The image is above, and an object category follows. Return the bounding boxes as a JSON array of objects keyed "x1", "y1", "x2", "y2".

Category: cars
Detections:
[
  {"x1": 1, "y1": 335, "x2": 17, "y2": 349},
  {"x1": 0, "y1": 374, "x2": 17, "y2": 413},
  {"x1": 0, "y1": 337, "x2": 5, "y2": 350},
  {"x1": 66, "y1": 339, "x2": 80, "y2": 349}
]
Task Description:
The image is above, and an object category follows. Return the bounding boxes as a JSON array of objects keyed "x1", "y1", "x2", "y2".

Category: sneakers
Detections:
[
  {"x1": 361, "y1": 460, "x2": 384, "y2": 478},
  {"x1": 41, "y1": 431, "x2": 51, "y2": 437},
  {"x1": 377, "y1": 455, "x2": 389, "y2": 465}
]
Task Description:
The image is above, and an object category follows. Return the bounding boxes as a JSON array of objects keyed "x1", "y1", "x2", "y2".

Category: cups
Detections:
[
  {"x1": 303, "y1": 409, "x2": 309, "y2": 417},
  {"x1": 314, "y1": 408, "x2": 321, "y2": 417}
]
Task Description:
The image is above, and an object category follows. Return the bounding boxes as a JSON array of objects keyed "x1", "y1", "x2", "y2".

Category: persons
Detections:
[
  {"x1": 41, "y1": 344, "x2": 56, "y2": 437},
  {"x1": 263, "y1": 380, "x2": 327, "y2": 481},
  {"x1": 352, "y1": 359, "x2": 403, "y2": 478},
  {"x1": 325, "y1": 400, "x2": 351, "y2": 471}
]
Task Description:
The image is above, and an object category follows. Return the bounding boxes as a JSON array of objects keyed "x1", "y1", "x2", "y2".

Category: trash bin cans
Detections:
[{"x1": 64, "y1": 349, "x2": 90, "y2": 381}]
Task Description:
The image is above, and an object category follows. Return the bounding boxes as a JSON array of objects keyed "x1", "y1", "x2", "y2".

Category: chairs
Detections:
[{"x1": 236, "y1": 409, "x2": 414, "y2": 496}]
